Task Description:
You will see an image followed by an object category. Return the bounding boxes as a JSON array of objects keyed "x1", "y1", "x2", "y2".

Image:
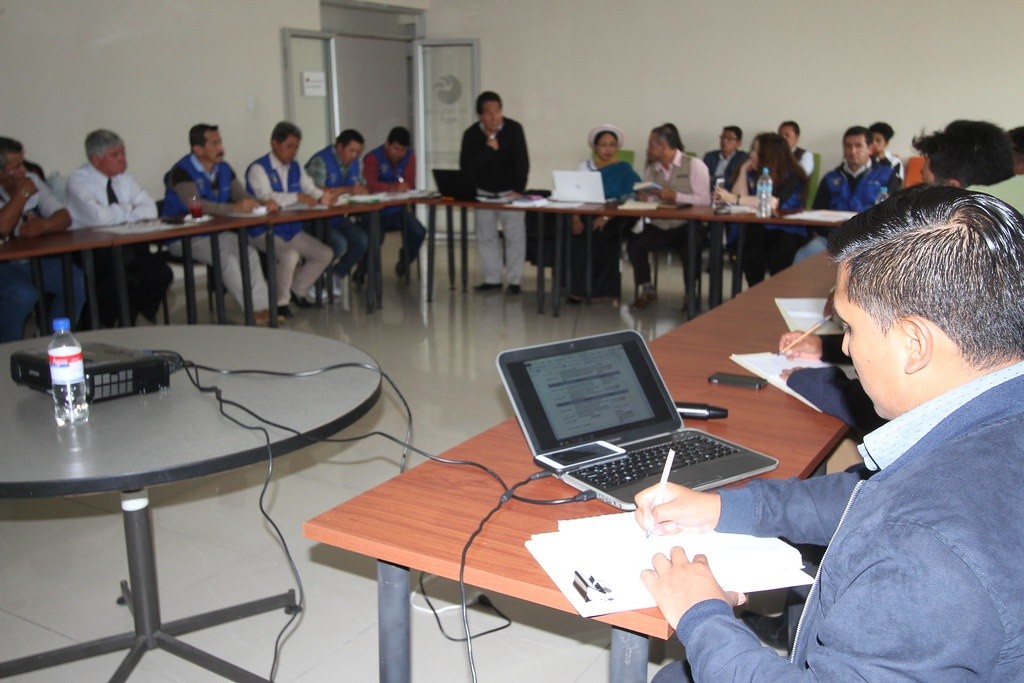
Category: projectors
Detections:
[{"x1": 9, "y1": 343, "x2": 171, "y2": 405}]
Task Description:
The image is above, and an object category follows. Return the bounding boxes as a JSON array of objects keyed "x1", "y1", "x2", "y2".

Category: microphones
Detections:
[{"x1": 674, "y1": 402, "x2": 728, "y2": 420}]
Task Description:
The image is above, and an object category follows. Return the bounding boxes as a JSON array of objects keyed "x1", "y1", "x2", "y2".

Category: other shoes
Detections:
[
  {"x1": 507, "y1": 284, "x2": 522, "y2": 296},
  {"x1": 290, "y1": 292, "x2": 318, "y2": 309},
  {"x1": 353, "y1": 272, "x2": 366, "y2": 284},
  {"x1": 275, "y1": 304, "x2": 293, "y2": 319},
  {"x1": 395, "y1": 258, "x2": 412, "y2": 289},
  {"x1": 631, "y1": 285, "x2": 658, "y2": 312},
  {"x1": 474, "y1": 282, "x2": 504, "y2": 291},
  {"x1": 253, "y1": 311, "x2": 285, "y2": 328},
  {"x1": 306, "y1": 284, "x2": 344, "y2": 298}
]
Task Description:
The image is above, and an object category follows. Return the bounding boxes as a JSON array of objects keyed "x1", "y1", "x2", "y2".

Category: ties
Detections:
[{"x1": 103, "y1": 180, "x2": 121, "y2": 204}]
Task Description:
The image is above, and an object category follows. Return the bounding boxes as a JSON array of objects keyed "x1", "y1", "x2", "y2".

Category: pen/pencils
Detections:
[
  {"x1": 646, "y1": 449, "x2": 676, "y2": 539},
  {"x1": 778, "y1": 314, "x2": 831, "y2": 357}
]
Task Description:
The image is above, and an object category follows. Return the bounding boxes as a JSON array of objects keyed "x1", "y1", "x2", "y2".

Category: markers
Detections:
[{"x1": 675, "y1": 402, "x2": 729, "y2": 421}]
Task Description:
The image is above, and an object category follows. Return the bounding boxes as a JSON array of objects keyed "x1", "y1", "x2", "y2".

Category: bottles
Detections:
[
  {"x1": 47, "y1": 318, "x2": 89, "y2": 427},
  {"x1": 756, "y1": 167, "x2": 773, "y2": 218},
  {"x1": 875, "y1": 187, "x2": 889, "y2": 204}
]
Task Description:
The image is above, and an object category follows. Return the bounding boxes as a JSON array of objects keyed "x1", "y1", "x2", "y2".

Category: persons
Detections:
[
  {"x1": 304, "y1": 129, "x2": 369, "y2": 298},
  {"x1": 631, "y1": 183, "x2": 1024, "y2": 683},
  {"x1": 0, "y1": 123, "x2": 284, "y2": 342},
  {"x1": 550, "y1": 120, "x2": 814, "y2": 319},
  {"x1": 793, "y1": 121, "x2": 905, "y2": 267},
  {"x1": 245, "y1": 121, "x2": 336, "y2": 319},
  {"x1": 460, "y1": 91, "x2": 529, "y2": 296},
  {"x1": 352, "y1": 127, "x2": 426, "y2": 284},
  {"x1": 912, "y1": 119, "x2": 1024, "y2": 219}
]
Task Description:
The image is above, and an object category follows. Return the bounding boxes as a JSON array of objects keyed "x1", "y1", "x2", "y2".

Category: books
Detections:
[
  {"x1": 92, "y1": 217, "x2": 197, "y2": 235},
  {"x1": 618, "y1": 197, "x2": 691, "y2": 210},
  {"x1": 730, "y1": 350, "x2": 861, "y2": 414},
  {"x1": 523, "y1": 504, "x2": 815, "y2": 621},
  {"x1": 775, "y1": 296, "x2": 849, "y2": 335},
  {"x1": 229, "y1": 188, "x2": 435, "y2": 215},
  {"x1": 783, "y1": 209, "x2": 858, "y2": 223},
  {"x1": 513, "y1": 197, "x2": 546, "y2": 208}
]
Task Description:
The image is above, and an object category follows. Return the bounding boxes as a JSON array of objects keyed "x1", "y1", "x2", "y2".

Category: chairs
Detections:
[
  {"x1": 804, "y1": 154, "x2": 821, "y2": 207},
  {"x1": 591, "y1": 148, "x2": 634, "y2": 169}
]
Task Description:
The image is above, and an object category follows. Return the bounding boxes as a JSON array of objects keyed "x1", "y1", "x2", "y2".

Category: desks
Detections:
[
  {"x1": 0, "y1": 321, "x2": 384, "y2": 683},
  {"x1": 0, "y1": 188, "x2": 848, "y2": 683}
]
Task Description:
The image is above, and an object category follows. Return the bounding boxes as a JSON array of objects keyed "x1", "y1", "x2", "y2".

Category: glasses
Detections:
[{"x1": 719, "y1": 134, "x2": 738, "y2": 141}]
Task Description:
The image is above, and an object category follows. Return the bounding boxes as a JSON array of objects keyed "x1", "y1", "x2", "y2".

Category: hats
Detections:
[{"x1": 588, "y1": 124, "x2": 624, "y2": 150}]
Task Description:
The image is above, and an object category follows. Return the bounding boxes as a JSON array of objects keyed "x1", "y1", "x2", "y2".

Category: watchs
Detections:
[
  {"x1": 18, "y1": 186, "x2": 30, "y2": 199},
  {"x1": 603, "y1": 215, "x2": 609, "y2": 221}
]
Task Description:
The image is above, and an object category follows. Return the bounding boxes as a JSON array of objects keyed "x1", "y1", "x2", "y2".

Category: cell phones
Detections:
[
  {"x1": 534, "y1": 440, "x2": 626, "y2": 475},
  {"x1": 707, "y1": 372, "x2": 768, "y2": 388},
  {"x1": 713, "y1": 178, "x2": 726, "y2": 208}
]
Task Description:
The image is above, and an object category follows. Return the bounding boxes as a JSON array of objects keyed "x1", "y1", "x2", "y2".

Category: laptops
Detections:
[
  {"x1": 553, "y1": 171, "x2": 616, "y2": 204},
  {"x1": 496, "y1": 329, "x2": 779, "y2": 510},
  {"x1": 431, "y1": 168, "x2": 500, "y2": 201}
]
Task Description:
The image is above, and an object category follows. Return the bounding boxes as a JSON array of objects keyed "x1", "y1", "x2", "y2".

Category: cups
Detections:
[{"x1": 188, "y1": 195, "x2": 202, "y2": 218}]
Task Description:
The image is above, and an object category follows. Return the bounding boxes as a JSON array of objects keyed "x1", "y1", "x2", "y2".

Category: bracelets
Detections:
[{"x1": 736, "y1": 195, "x2": 740, "y2": 204}]
